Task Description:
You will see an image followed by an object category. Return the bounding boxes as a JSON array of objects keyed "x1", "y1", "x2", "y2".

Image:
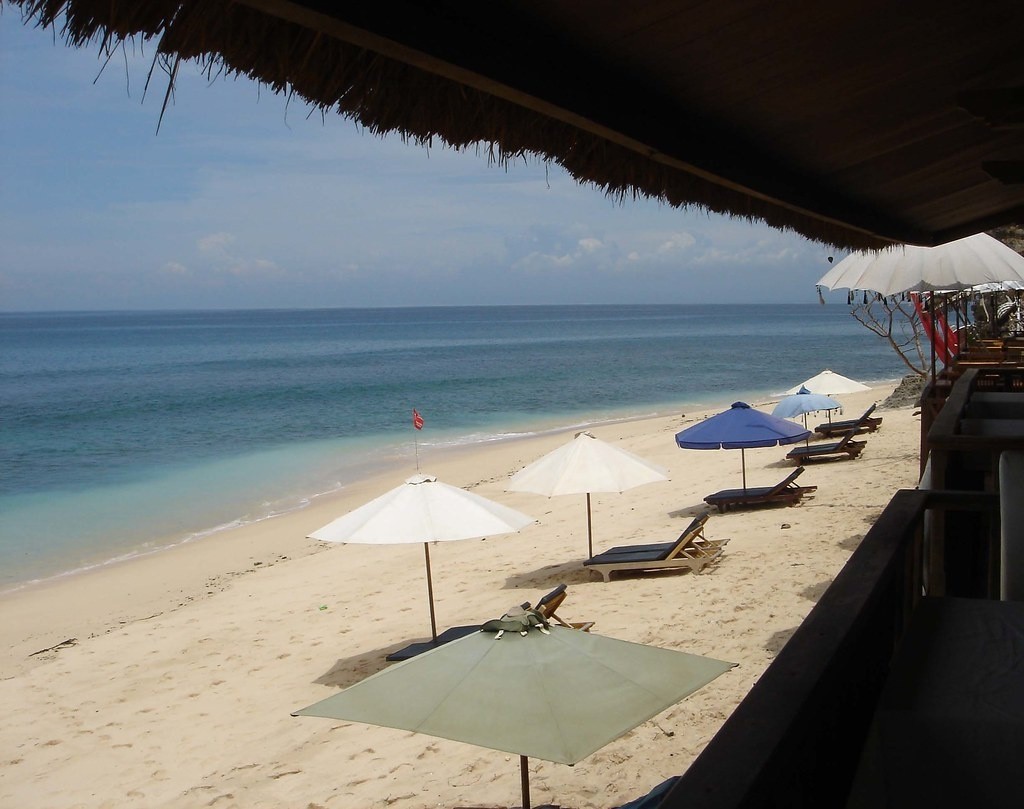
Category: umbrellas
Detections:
[
  {"x1": 306, "y1": 476, "x2": 534, "y2": 641},
  {"x1": 815, "y1": 233, "x2": 1024, "y2": 383},
  {"x1": 502, "y1": 433, "x2": 667, "y2": 559},
  {"x1": 771, "y1": 371, "x2": 872, "y2": 450},
  {"x1": 675, "y1": 402, "x2": 811, "y2": 490}
]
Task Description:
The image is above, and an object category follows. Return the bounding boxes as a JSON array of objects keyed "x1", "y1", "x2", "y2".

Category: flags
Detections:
[{"x1": 413, "y1": 408, "x2": 424, "y2": 431}]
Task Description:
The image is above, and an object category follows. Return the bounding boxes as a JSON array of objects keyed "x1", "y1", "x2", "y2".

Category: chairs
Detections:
[
  {"x1": 703, "y1": 465, "x2": 818, "y2": 514},
  {"x1": 385, "y1": 584, "x2": 596, "y2": 662},
  {"x1": 786, "y1": 426, "x2": 868, "y2": 467},
  {"x1": 583, "y1": 510, "x2": 731, "y2": 583},
  {"x1": 815, "y1": 402, "x2": 883, "y2": 439}
]
